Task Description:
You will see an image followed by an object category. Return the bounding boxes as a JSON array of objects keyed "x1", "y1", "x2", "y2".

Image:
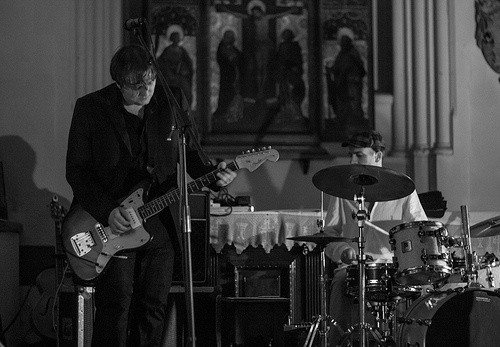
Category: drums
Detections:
[
  {"x1": 344, "y1": 263, "x2": 423, "y2": 301},
  {"x1": 397, "y1": 288, "x2": 500, "y2": 347},
  {"x1": 389, "y1": 220, "x2": 453, "y2": 285}
]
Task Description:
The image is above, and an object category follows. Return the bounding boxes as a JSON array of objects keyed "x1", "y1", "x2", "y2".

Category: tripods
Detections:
[{"x1": 304, "y1": 190, "x2": 388, "y2": 347}]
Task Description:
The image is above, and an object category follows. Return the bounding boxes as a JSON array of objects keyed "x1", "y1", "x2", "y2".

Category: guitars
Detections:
[{"x1": 61, "y1": 145, "x2": 280, "y2": 281}]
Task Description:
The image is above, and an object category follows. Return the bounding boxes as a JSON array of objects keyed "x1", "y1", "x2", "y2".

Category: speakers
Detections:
[{"x1": 74, "y1": 188, "x2": 222, "y2": 347}]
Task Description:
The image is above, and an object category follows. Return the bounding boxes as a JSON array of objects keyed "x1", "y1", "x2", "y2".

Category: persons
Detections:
[
  {"x1": 322, "y1": 131, "x2": 448, "y2": 347},
  {"x1": 66, "y1": 44, "x2": 237, "y2": 347}
]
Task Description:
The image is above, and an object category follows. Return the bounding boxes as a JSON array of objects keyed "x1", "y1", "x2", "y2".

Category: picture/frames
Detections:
[{"x1": 122, "y1": 0, "x2": 377, "y2": 158}]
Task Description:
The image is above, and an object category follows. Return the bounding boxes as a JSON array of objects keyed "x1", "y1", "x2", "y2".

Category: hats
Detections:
[{"x1": 340, "y1": 133, "x2": 386, "y2": 151}]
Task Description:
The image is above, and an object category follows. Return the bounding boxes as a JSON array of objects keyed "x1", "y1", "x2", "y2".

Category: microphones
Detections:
[{"x1": 124, "y1": 17, "x2": 146, "y2": 30}]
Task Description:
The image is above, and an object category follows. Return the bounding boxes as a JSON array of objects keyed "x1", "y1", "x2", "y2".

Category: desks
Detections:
[{"x1": 210, "y1": 209, "x2": 327, "y2": 347}]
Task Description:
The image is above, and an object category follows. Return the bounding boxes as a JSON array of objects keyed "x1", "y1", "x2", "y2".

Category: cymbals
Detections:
[
  {"x1": 470, "y1": 215, "x2": 500, "y2": 238},
  {"x1": 285, "y1": 232, "x2": 356, "y2": 245},
  {"x1": 311, "y1": 164, "x2": 416, "y2": 204}
]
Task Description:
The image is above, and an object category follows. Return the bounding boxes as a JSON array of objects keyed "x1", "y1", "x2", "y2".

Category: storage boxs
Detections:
[{"x1": 56, "y1": 286, "x2": 95, "y2": 347}]
